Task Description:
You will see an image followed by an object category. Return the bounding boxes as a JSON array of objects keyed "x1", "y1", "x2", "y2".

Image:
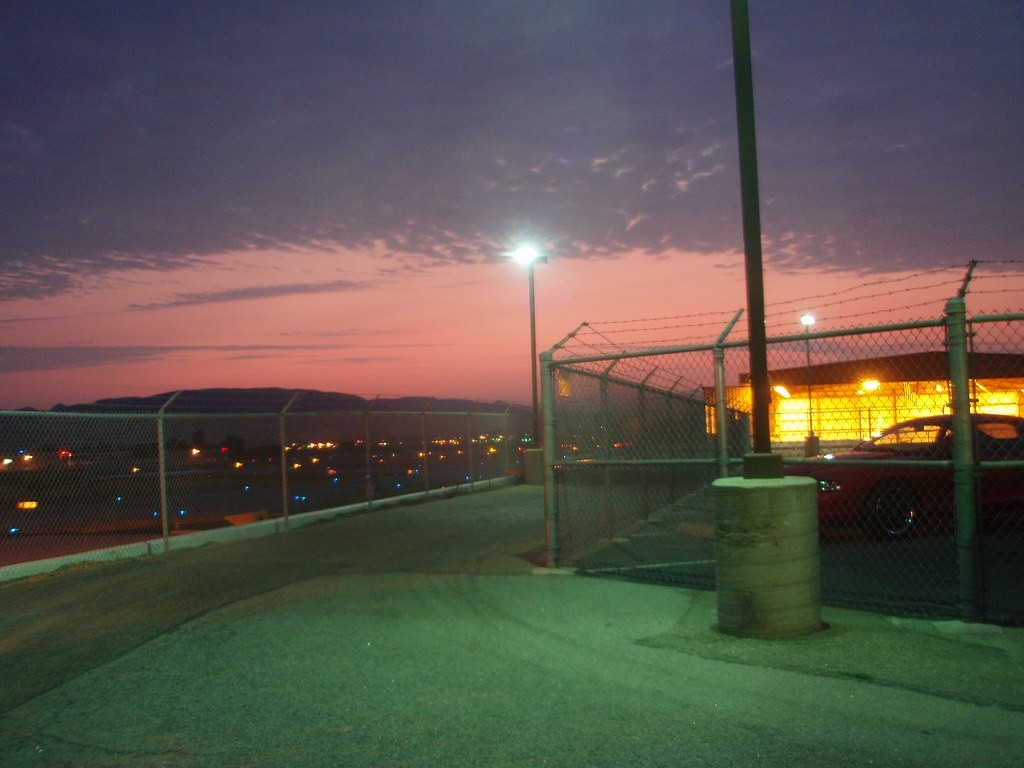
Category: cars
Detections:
[{"x1": 779, "y1": 412, "x2": 1024, "y2": 542}]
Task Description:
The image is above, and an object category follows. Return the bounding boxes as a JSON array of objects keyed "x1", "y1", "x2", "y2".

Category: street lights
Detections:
[
  {"x1": 800, "y1": 313, "x2": 815, "y2": 436},
  {"x1": 525, "y1": 253, "x2": 548, "y2": 444},
  {"x1": 863, "y1": 379, "x2": 880, "y2": 438}
]
